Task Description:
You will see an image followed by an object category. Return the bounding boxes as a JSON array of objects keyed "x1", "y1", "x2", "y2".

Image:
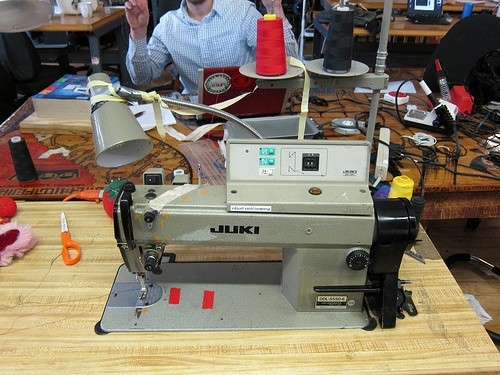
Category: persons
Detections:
[{"x1": 125, "y1": 0, "x2": 299, "y2": 94}]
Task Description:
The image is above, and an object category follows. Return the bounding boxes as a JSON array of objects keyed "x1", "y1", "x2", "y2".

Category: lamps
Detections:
[{"x1": 86, "y1": 72, "x2": 265, "y2": 170}]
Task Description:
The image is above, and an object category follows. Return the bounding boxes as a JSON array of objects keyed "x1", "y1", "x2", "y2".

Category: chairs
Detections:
[{"x1": 0, "y1": 32, "x2": 119, "y2": 124}]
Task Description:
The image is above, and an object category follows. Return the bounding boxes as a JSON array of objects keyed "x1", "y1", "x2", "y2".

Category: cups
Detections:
[
  {"x1": 461, "y1": 3, "x2": 473, "y2": 19},
  {"x1": 79, "y1": 2, "x2": 93, "y2": 18}
]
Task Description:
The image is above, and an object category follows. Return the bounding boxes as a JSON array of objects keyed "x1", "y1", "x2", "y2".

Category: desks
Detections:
[
  {"x1": 25, "y1": 6, "x2": 132, "y2": 88},
  {"x1": 321, "y1": 0, "x2": 499, "y2": 16},
  {"x1": 0, "y1": 94, "x2": 500, "y2": 234},
  {"x1": 312, "y1": 11, "x2": 465, "y2": 73}
]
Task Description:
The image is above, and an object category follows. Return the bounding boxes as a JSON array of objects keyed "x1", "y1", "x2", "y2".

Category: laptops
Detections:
[
  {"x1": 163, "y1": 65, "x2": 290, "y2": 121},
  {"x1": 407, "y1": 0, "x2": 450, "y2": 24}
]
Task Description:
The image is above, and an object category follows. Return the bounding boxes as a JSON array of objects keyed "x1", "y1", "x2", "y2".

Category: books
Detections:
[{"x1": 32, "y1": 74, "x2": 120, "y2": 120}]
